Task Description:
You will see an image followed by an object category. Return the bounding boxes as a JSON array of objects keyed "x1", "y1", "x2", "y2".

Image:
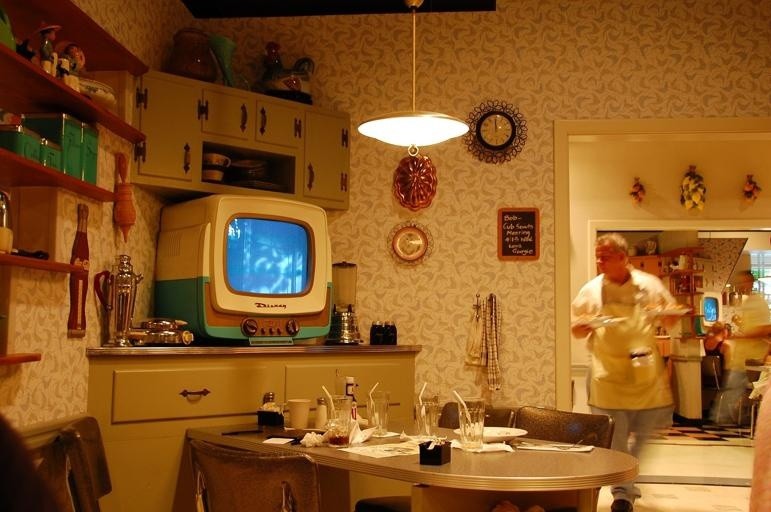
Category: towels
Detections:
[{"x1": 463, "y1": 290, "x2": 503, "y2": 391}]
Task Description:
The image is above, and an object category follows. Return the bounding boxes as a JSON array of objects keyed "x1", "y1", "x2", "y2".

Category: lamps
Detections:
[{"x1": 357, "y1": 0, "x2": 470, "y2": 157}]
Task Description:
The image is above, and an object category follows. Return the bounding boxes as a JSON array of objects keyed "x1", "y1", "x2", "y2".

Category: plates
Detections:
[
  {"x1": 454, "y1": 425, "x2": 529, "y2": 443},
  {"x1": 643, "y1": 308, "x2": 691, "y2": 317}
]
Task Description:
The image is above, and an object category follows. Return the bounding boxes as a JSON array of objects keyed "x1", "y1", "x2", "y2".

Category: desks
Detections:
[{"x1": 183, "y1": 418, "x2": 637, "y2": 511}]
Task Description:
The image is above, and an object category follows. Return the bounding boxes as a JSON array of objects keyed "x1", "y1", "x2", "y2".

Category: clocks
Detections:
[{"x1": 462, "y1": 98, "x2": 528, "y2": 165}]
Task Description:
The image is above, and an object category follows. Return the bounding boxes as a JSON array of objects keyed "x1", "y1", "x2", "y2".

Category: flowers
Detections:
[{"x1": 629, "y1": 164, "x2": 761, "y2": 211}]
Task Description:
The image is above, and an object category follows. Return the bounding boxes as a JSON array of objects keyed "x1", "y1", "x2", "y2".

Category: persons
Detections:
[
  {"x1": 572, "y1": 233, "x2": 688, "y2": 512},
  {"x1": 40, "y1": 27, "x2": 58, "y2": 77},
  {"x1": 701, "y1": 271, "x2": 771, "y2": 428},
  {"x1": 703, "y1": 321, "x2": 731, "y2": 387}
]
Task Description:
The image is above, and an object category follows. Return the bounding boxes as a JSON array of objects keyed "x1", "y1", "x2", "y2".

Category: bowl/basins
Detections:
[
  {"x1": 234, "y1": 159, "x2": 268, "y2": 180},
  {"x1": 570, "y1": 317, "x2": 621, "y2": 328}
]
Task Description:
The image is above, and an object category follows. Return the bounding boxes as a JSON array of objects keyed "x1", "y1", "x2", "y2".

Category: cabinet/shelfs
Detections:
[
  {"x1": 0, "y1": 0, "x2": 149, "y2": 366},
  {"x1": 85, "y1": 345, "x2": 422, "y2": 512},
  {"x1": 628, "y1": 246, "x2": 718, "y2": 428},
  {"x1": 132, "y1": 69, "x2": 349, "y2": 209}
]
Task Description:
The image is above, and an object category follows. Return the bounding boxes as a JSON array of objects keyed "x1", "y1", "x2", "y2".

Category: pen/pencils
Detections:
[{"x1": 221, "y1": 430, "x2": 264, "y2": 435}]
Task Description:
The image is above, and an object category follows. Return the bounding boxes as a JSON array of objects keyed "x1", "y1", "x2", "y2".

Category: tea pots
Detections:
[{"x1": 94, "y1": 253, "x2": 143, "y2": 349}]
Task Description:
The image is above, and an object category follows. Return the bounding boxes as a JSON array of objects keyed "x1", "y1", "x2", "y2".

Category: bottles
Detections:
[
  {"x1": 66, "y1": 203, "x2": 89, "y2": 338},
  {"x1": 370, "y1": 319, "x2": 396, "y2": 344},
  {"x1": 315, "y1": 376, "x2": 357, "y2": 430},
  {"x1": 679, "y1": 251, "x2": 688, "y2": 270}
]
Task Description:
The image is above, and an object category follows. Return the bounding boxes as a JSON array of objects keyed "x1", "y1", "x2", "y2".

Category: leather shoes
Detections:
[{"x1": 611, "y1": 499, "x2": 633, "y2": 512}]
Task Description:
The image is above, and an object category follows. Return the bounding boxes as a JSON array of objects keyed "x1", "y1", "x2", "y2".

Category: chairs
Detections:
[
  {"x1": 512, "y1": 404, "x2": 618, "y2": 512},
  {"x1": 703, "y1": 356, "x2": 738, "y2": 425},
  {"x1": 187, "y1": 435, "x2": 324, "y2": 512},
  {"x1": 738, "y1": 359, "x2": 765, "y2": 438},
  {"x1": 435, "y1": 404, "x2": 521, "y2": 511}
]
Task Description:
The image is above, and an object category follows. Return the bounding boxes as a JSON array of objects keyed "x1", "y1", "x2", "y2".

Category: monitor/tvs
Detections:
[
  {"x1": 157, "y1": 196, "x2": 333, "y2": 345},
  {"x1": 702, "y1": 292, "x2": 724, "y2": 328}
]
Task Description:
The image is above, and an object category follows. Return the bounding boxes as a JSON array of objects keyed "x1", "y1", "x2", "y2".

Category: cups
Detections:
[
  {"x1": 325, "y1": 393, "x2": 487, "y2": 452},
  {"x1": 203, "y1": 152, "x2": 232, "y2": 183},
  {"x1": 287, "y1": 399, "x2": 312, "y2": 430}
]
